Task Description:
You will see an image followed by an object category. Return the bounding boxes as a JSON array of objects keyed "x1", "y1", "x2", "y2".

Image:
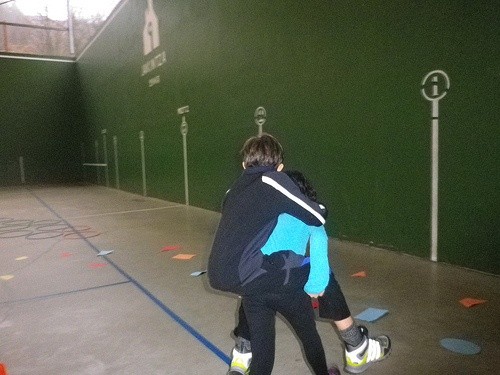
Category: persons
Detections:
[
  {"x1": 248, "y1": 169, "x2": 341, "y2": 375},
  {"x1": 208, "y1": 132, "x2": 393, "y2": 375}
]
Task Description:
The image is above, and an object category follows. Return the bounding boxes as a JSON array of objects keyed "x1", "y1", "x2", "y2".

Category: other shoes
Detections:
[{"x1": 328, "y1": 367, "x2": 340, "y2": 375}]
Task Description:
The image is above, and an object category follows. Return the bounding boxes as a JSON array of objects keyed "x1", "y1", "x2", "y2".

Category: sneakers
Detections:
[
  {"x1": 344, "y1": 324, "x2": 392, "y2": 374},
  {"x1": 226, "y1": 345, "x2": 252, "y2": 375}
]
